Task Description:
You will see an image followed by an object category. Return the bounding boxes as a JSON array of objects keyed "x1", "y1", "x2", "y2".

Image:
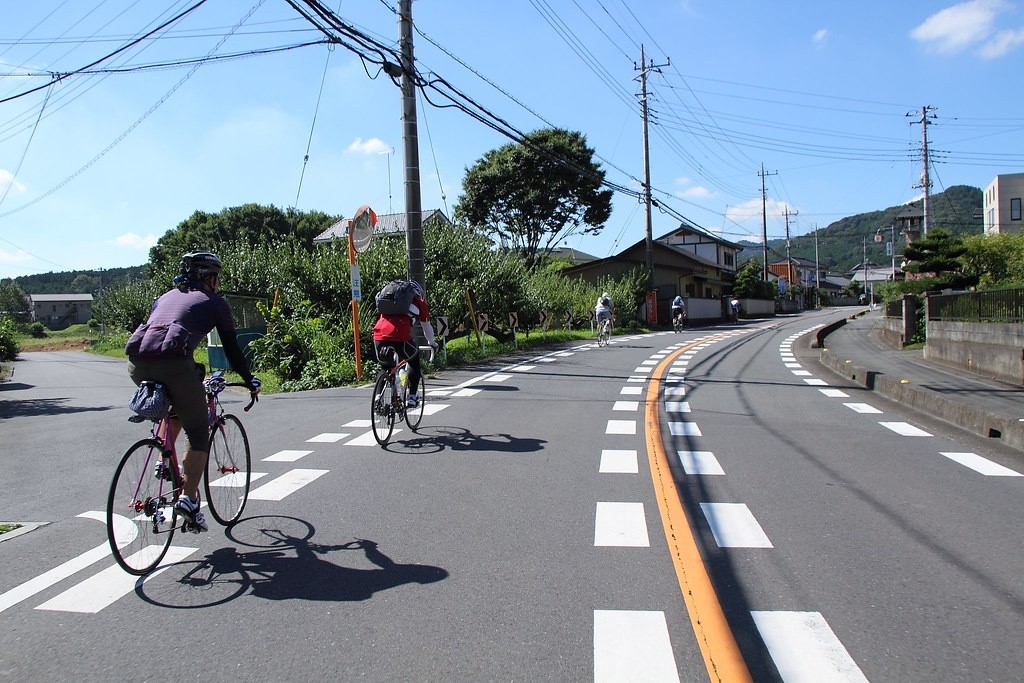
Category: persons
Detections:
[
  {"x1": 731, "y1": 297, "x2": 742, "y2": 321},
  {"x1": 373, "y1": 279, "x2": 439, "y2": 416},
  {"x1": 124, "y1": 250, "x2": 259, "y2": 532},
  {"x1": 595, "y1": 292, "x2": 617, "y2": 342},
  {"x1": 672, "y1": 296, "x2": 687, "y2": 327}
]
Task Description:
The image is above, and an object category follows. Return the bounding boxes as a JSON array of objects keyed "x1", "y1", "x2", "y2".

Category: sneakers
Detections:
[
  {"x1": 173, "y1": 494, "x2": 208, "y2": 533},
  {"x1": 407, "y1": 397, "x2": 419, "y2": 408},
  {"x1": 154, "y1": 460, "x2": 183, "y2": 479},
  {"x1": 375, "y1": 393, "x2": 384, "y2": 415}
]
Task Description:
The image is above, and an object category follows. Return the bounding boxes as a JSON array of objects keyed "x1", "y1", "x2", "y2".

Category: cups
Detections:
[{"x1": 399, "y1": 369, "x2": 407, "y2": 387}]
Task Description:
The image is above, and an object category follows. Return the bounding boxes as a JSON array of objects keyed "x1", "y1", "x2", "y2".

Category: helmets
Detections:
[
  {"x1": 409, "y1": 279, "x2": 425, "y2": 298},
  {"x1": 676, "y1": 295, "x2": 682, "y2": 299},
  {"x1": 179, "y1": 249, "x2": 222, "y2": 274},
  {"x1": 602, "y1": 292, "x2": 610, "y2": 297}
]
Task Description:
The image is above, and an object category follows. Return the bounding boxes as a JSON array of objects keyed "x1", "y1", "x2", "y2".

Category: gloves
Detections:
[{"x1": 430, "y1": 341, "x2": 439, "y2": 355}]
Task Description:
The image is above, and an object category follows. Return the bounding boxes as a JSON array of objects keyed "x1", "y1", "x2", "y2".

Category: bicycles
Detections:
[
  {"x1": 597, "y1": 314, "x2": 618, "y2": 348},
  {"x1": 370, "y1": 345, "x2": 441, "y2": 446},
  {"x1": 732, "y1": 306, "x2": 743, "y2": 325},
  {"x1": 673, "y1": 312, "x2": 687, "y2": 334},
  {"x1": 106, "y1": 375, "x2": 260, "y2": 577}
]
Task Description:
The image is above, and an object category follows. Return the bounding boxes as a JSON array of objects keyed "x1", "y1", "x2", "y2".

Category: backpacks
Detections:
[
  {"x1": 599, "y1": 296, "x2": 610, "y2": 308},
  {"x1": 674, "y1": 297, "x2": 682, "y2": 307},
  {"x1": 375, "y1": 279, "x2": 420, "y2": 316}
]
